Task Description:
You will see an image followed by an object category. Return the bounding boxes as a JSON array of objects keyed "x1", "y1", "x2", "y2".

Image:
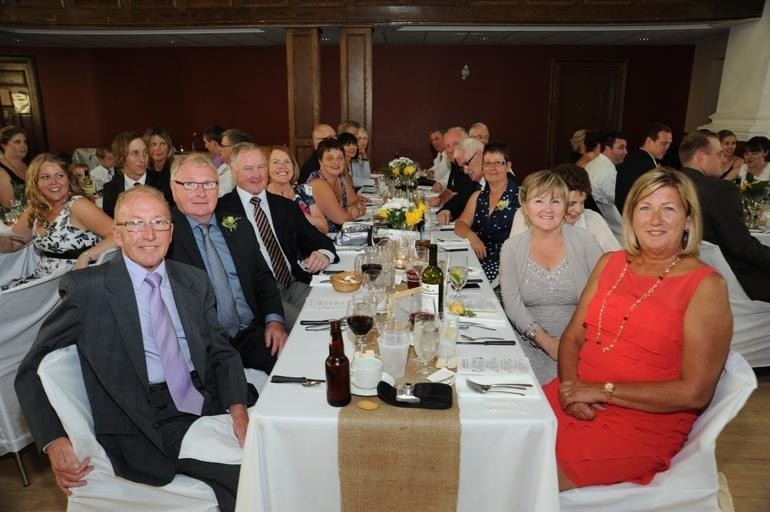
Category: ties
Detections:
[
  {"x1": 146, "y1": 272, "x2": 205, "y2": 417},
  {"x1": 250, "y1": 196, "x2": 292, "y2": 289},
  {"x1": 197, "y1": 223, "x2": 241, "y2": 339}
]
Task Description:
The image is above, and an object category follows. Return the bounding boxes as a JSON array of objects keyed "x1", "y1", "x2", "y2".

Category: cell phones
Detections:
[{"x1": 451, "y1": 283, "x2": 480, "y2": 290}]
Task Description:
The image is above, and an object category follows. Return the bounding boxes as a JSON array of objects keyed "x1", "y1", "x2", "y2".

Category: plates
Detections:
[
  {"x1": 352, "y1": 370, "x2": 396, "y2": 396},
  {"x1": 748, "y1": 229, "x2": 764, "y2": 233}
]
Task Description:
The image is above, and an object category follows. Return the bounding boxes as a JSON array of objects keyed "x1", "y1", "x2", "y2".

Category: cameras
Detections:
[{"x1": 396, "y1": 383, "x2": 421, "y2": 403}]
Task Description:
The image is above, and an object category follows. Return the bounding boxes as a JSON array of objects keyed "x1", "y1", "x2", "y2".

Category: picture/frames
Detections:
[
  {"x1": 544, "y1": 58, "x2": 630, "y2": 169},
  {"x1": 0, "y1": 54, "x2": 50, "y2": 164}
]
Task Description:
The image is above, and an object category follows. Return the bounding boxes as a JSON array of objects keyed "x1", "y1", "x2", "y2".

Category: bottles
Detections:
[
  {"x1": 320, "y1": 320, "x2": 352, "y2": 409},
  {"x1": 422, "y1": 245, "x2": 444, "y2": 314},
  {"x1": 405, "y1": 249, "x2": 420, "y2": 289}
]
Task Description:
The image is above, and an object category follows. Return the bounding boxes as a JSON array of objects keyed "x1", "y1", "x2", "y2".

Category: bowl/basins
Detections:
[{"x1": 330, "y1": 270, "x2": 364, "y2": 293}]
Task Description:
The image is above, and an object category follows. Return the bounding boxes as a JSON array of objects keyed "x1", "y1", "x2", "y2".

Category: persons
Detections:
[
  {"x1": 12, "y1": 188, "x2": 253, "y2": 512},
  {"x1": 534, "y1": 165, "x2": 735, "y2": 493},
  {"x1": 2, "y1": 118, "x2": 769, "y2": 408}
]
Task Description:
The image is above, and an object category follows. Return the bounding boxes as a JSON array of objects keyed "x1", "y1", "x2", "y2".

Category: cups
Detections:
[
  {"x1": 353, "y1": 244, "x2": 397, "y2": 290},
  {"x1": 412, "y1": 312, "x2": 438, "y2": 376},
  {"x1": 371, "y1": 205, "x2": 390, "y2": 246},
  {"x1": 378, "y1": 176, "x2": 386, "y2": 194},
  {"x1": 412, "y1": 191, "x2": 426, "y2": 207},
  {"x1": 4, "y1": 200, "x2": 24, "y2": 226},
  {"x1": 349, "y1": 355, "x2": 380, "y2": 390},
  {"x1": 372, "y1": 313, "x2": 409, "y2": 377},
  {"x1": 435, "y1": 310, "x2": 461, "y2": 359}
]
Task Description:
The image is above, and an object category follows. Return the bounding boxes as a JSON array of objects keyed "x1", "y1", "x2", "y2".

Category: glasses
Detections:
[
  {"x1": 116, "y1": 219, "x2": 173, "y2": 234},
  {"x1": 483, "y1": 161, "x2": 506, "y2": 167},
  {"x1": 173, "y1": 181, "x2": 221, "y2": 191},
  {"x1": 464, "y1": 151, "x2": 478, "y2": 166}
]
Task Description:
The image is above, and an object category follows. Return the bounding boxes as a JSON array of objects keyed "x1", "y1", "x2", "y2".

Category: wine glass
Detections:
[
  {"x1": 446, "y1": 254, "x2": 470, "y2": 301},
  {"x1": 744, "y1": 190, "x2": 766, "y2": 230},
  {"x1": 345, "y1": 297, "x2": 375, "y2": 354},
  {"x1": 391, "y1": 176, "x2": 419, "y2": 199}
]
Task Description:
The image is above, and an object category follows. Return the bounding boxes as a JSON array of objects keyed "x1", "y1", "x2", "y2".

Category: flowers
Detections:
[{"x1": 388, "y1": 157, "x2": 419, "y2": 178}]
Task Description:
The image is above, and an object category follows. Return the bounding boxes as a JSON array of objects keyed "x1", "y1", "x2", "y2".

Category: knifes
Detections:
[{"x1": 451, "y1": 341, "x2": 519, "y2": 348}]
